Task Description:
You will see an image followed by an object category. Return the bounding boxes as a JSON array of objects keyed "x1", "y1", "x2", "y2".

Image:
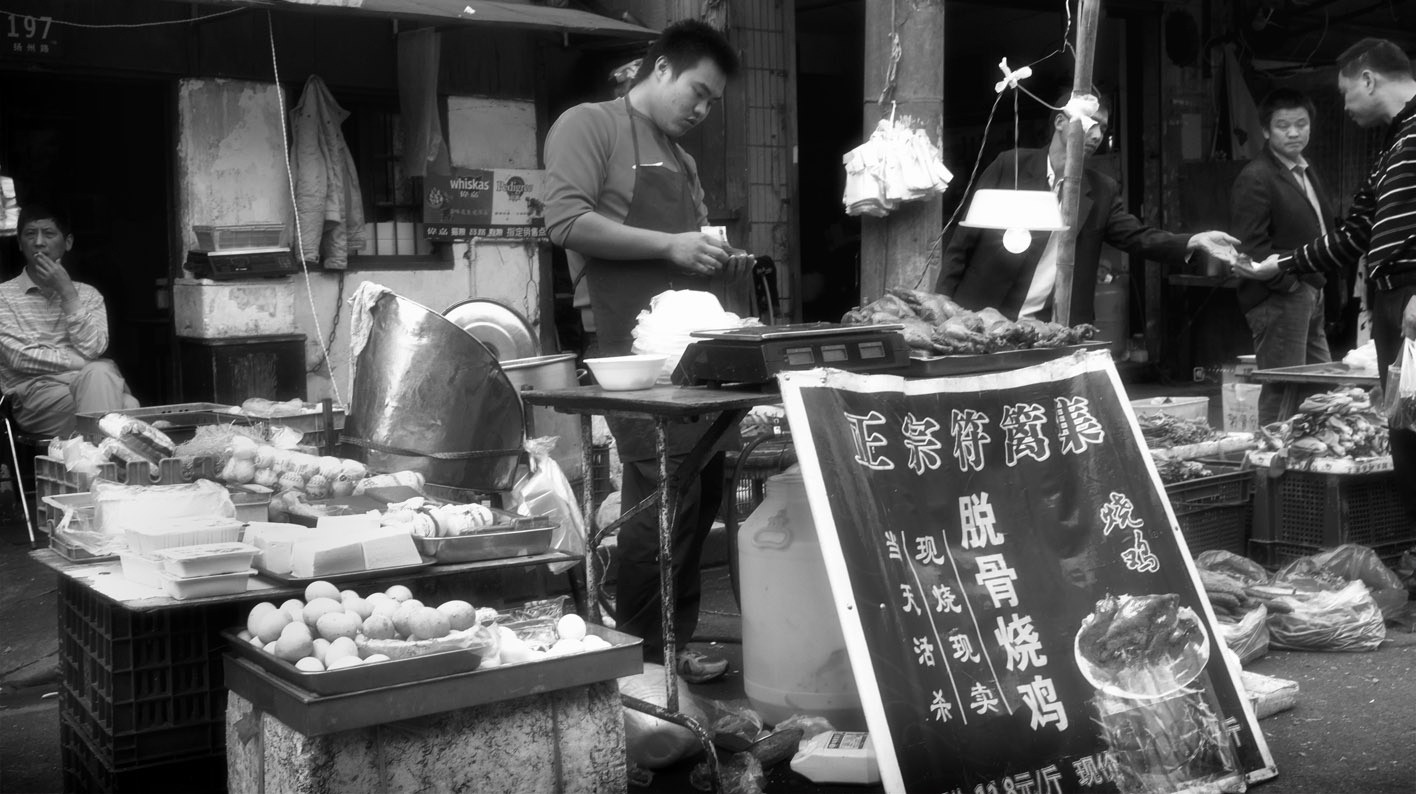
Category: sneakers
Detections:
[{"x1": 674, "y1": 648, "x2": 727, "y2": 681}]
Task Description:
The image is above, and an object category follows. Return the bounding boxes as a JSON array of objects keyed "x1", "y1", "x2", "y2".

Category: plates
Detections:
[{"x1": 1075, "y1": 604, "x2": 1210, "y2": 699}]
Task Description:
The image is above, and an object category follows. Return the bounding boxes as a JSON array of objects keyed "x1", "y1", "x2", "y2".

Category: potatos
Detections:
[
  {"x1": 239, "y1": 579, "x2": 613, "y2": 671},
  {"x1": 218, "y1": 436, "x2": 363, "y2": 499}
]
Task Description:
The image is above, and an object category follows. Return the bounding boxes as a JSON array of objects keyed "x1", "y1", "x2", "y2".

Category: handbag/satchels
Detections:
[{"x1": 1382, "y1": 338, "x2": 1416, "y2": 433}]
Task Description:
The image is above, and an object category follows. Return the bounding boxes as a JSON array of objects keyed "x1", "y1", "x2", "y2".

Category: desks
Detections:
[
  {"x1": 23, "y1": 533, "x2": 589, "y2": 616},
  {"x1": 518, "y1": 389, "x2": 784, "y2": 794},
  {"x1": 1250, "y1": 362, "x2": 1381, "y2": 422}
]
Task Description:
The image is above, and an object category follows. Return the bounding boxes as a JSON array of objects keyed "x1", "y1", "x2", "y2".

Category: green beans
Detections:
[{"x1": 1265, "y1": 602, "x2": 1386, "y2": 651}]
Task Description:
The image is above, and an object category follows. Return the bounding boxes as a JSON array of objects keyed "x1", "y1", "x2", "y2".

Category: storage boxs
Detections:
[
  {"x1": 409, "y1": 164, "x2": 545, "y2": 239},
  {"x1": 36, "y1": 401, "x2": 347, "y2": 794},
  {"x1": 175, "y1": 277, "x2": 300, "y2": 341},
  {"x1": 1129, "y1": 383, "x2": 1416, "y2": 569}
]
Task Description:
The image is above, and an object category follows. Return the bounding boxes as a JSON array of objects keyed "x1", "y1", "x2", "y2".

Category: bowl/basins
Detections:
[{"x1": 583, "y1": 354, "x2": 670, "y2": 391}]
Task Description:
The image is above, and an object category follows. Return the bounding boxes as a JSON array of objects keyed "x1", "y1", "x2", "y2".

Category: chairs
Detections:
[{"x1": 0, "y1": 394, "x2": 54, "y2": 549}]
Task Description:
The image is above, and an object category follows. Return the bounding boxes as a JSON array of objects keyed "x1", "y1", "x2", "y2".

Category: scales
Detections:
[
  {"x1": 1234, "y1": 365, "x2": 1262, "y2": 385},
  {"x1": 669, "y1": 321, "x2": 910, "y2": 388},
  {"x1": 184, "y1": 247, "x2": 299, "y2": 282}
]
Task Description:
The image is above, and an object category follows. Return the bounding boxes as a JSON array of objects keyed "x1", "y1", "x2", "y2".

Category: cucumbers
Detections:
[{"x1": 1202, "y1": 581, "x2": 1294, "y2": 623}]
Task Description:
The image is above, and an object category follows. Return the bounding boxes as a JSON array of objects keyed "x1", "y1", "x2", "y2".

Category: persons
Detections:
[
  {"x1": 543, "y1": 20, "x2": 741, "y2": 681},
  {"x1": 0, "y1": 206, "x2": 139, "y2": 440},
  {"x1": 1232, "y1": 82, "x2": 1335, "y2": 430},
  {"x1": 934, "y1": 93, "x2": 1242, "y2": 328},
  {"x1": 1231, "y1": 37, "x2": 1416, "y2": 599}
]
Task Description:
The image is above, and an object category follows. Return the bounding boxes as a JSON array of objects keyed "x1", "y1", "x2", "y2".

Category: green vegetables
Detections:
[{"x1": 1248, "y1": 385, "x2": 1392, "y2": 477}]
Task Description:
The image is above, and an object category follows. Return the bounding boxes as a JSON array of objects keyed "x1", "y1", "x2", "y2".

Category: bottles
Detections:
[{"x1": 789, "y1": 731, "x2": 880, "y2": 784}]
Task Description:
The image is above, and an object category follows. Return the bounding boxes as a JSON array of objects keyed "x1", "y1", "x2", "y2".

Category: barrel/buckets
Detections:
[
  {"x1": 498, "y1": 353, "x2": 587, "y2": 482},
  {"x1": 1127, "y1": 395, "x2": 1209, "y2": 422},
  {"x1": 736, "y1": 473, "x2": 869, "y2": 733}
]
